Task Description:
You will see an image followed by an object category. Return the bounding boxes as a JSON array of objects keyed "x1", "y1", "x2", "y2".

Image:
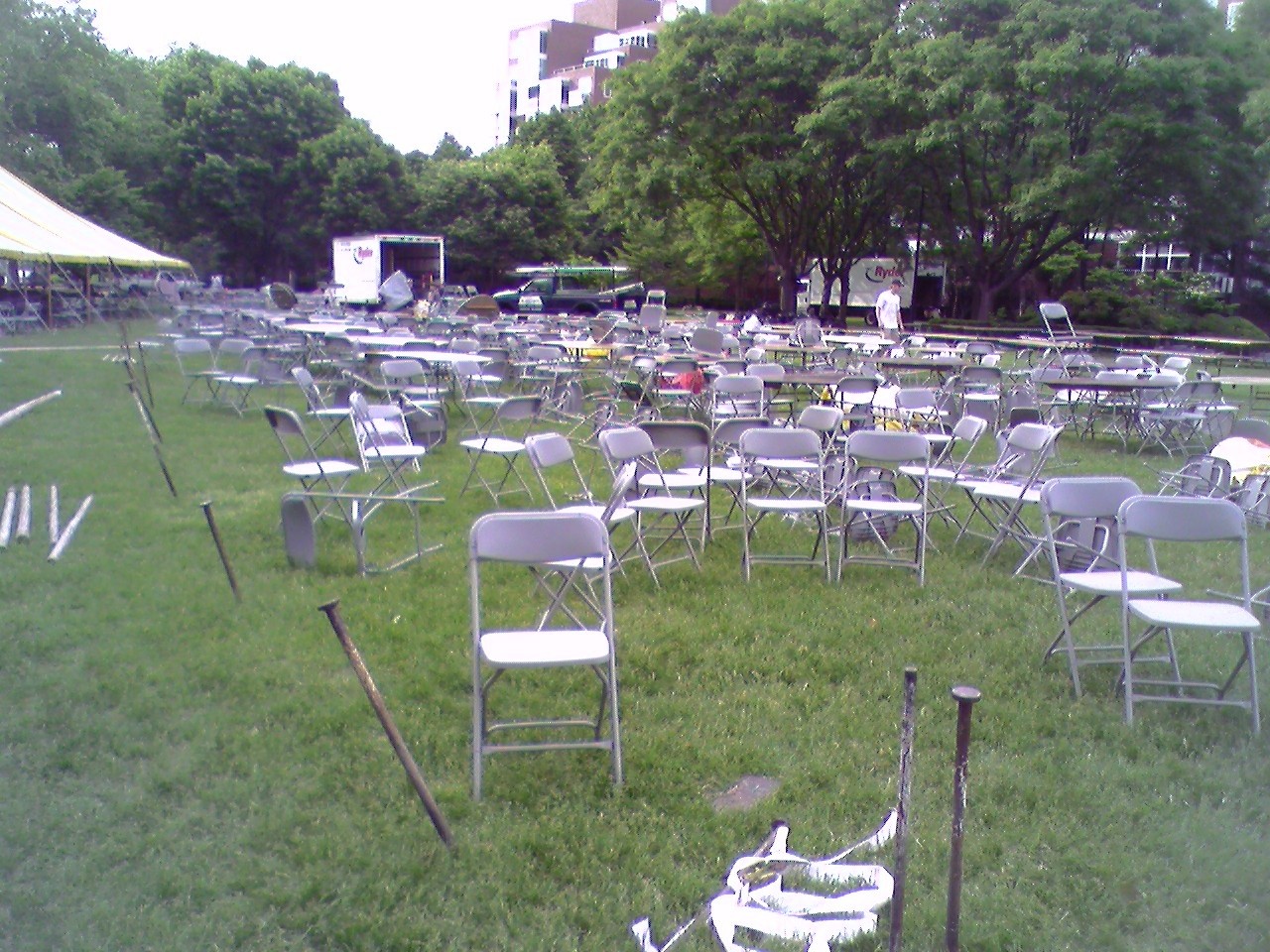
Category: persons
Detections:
[{"x1": 876, "y1": 279, "x2": 904, "y2": 341}]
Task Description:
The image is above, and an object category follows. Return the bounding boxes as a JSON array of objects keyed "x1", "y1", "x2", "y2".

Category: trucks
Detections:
[
  {"x1": 773, "y1": 256, "x2": 947, "y2": 328},
  {"x1": 329, "y1": 233, "x2": 446, "y2": 309}
]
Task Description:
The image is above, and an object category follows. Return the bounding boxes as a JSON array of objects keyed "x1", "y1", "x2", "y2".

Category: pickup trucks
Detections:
[{"x1": 493, "y1": 272, "x2": 618, "y2": 317}]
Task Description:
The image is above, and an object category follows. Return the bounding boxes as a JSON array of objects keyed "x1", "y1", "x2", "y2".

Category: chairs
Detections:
[{"x1": 0, "y1": 271, "x2": 1270, "y2": 804}]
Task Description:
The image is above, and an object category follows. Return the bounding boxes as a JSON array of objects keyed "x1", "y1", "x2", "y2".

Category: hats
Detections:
[{"x1": 892, "y1": 280, "x2": 904, "y2": 287}]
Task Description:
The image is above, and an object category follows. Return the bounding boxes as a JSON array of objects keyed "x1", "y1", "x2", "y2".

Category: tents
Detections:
[{"x1": 0, "y1": 165, "x2": 191, "y2": 270}]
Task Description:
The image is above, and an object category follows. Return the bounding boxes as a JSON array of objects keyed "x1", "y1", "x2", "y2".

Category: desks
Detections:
[
  {"x1": 284, "y1": 324, "x2": 383, "y2": 372},
  {"x1": 996, "y1": 337, "x2": 1091, "y2": 371},
  {"x1": 865, "y1": 357, "x2": 958, "y2": 387},
  {"x1": 764, "y1": 346, "x2": 836, "y2": 370},
  {"x1": 340, "y1": 333, "x2": 444, "y2": 352},
  {"x1": 369, "y1": 351, "x2": 494, "y2": 419},
  {"x1": 1037, "y1": 377, "x2": 1179, "y2": 450},
  {"x1": 764, "y1": 371, "x2": 856, "y2": 430}
]
